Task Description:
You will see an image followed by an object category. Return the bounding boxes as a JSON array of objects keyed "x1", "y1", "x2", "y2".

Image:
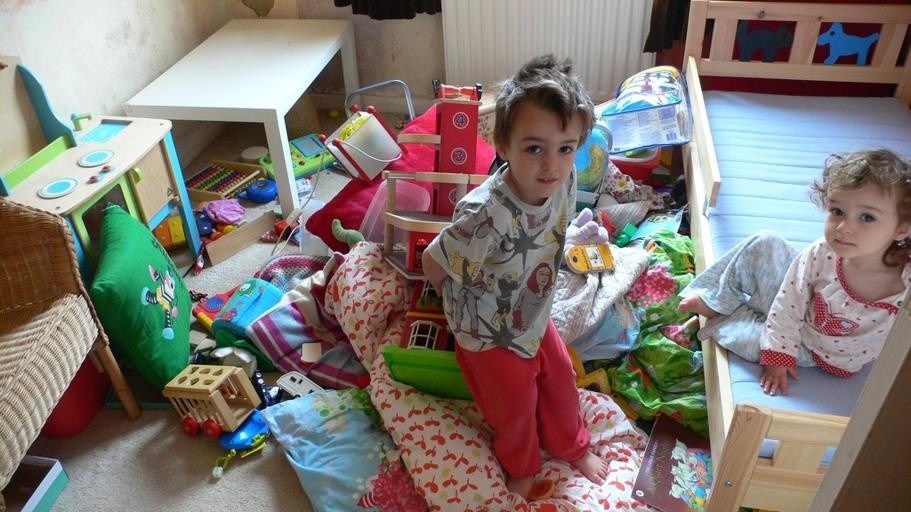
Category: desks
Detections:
[
  {"x1": 0, "y1": 55, "x2": 203, "y2": 291},
  {"x1": 122, "y1": 18, "x2": 361, "y2": 219}
]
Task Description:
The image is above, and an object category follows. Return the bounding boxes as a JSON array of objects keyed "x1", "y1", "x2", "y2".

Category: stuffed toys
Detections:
[{"x1": 559, "y1": 206, "x2": 609, "y2": 265}]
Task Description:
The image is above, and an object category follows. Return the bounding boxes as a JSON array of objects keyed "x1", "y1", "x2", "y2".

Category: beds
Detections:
[{"x1": 682, "y1": 0, "x2": 911, "y2": 512}]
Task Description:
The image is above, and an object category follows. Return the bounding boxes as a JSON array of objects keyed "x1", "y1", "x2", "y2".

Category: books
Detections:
[{"x1": 563, "y1": 243, "x2": 617, "y2": 274}]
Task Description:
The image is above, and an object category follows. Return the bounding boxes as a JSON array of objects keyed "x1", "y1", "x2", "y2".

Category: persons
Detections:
[
  {"x1": 676, "y1": 146, "x2": 910, "y2": 399},
  {"x1": 422, "y1": 52, "x2": 612, "y2": 500}
]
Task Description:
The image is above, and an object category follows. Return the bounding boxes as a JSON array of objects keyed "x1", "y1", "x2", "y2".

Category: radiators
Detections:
[{"x1": 441, "y1": 0, "x2": 658, "y2": 101}]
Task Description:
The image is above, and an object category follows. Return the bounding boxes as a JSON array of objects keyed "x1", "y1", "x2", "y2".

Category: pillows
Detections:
[{"x1": 89, "y1": 203, "x2": 193, "y2": 390}]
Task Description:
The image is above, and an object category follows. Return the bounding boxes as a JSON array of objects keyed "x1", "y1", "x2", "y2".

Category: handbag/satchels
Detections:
[{"x1": 602, "y1": 66, "x2": 692, "y2": 153}]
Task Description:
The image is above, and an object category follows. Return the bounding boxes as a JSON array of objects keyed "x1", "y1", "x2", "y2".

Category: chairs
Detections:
[{"x1": 0, "y1": 198, "x2": 140, "y2": 512}]
